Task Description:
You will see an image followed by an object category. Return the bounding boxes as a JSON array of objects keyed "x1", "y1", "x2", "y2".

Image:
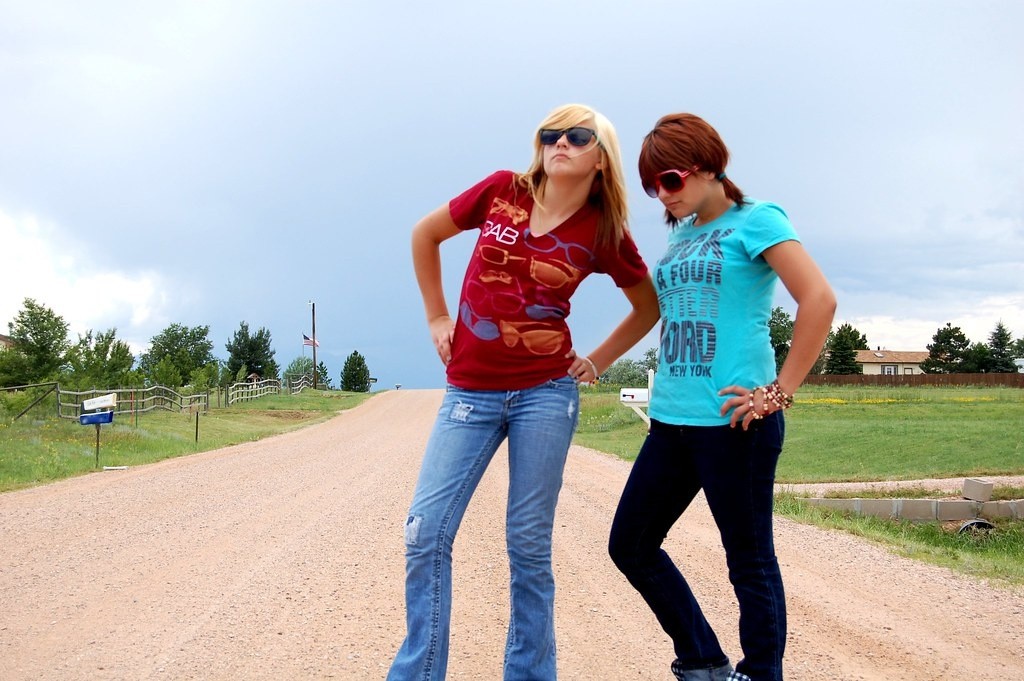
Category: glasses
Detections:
[
  {"x1": 539, "y1": 127, "x2": 598, "y2": 147},
  {"x1": 641, "y1": 161, "x2": 701, "y2": 198}
]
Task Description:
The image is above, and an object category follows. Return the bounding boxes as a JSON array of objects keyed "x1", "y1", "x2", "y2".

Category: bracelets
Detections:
[
  {"x1": 748, "y1": 379, "x2": 794, "y2": 421},
  {"x1": 582, "y1": 356, "x2": 600, "y2": 386}
]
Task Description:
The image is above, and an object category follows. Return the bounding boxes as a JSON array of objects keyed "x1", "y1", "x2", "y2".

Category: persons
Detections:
[
  {"x1": 386, "y1": 102, "x2": 661, "y2": 681},
  {"x1": 607, "y1": 112, "x2": 839, "y2": 681}
]
